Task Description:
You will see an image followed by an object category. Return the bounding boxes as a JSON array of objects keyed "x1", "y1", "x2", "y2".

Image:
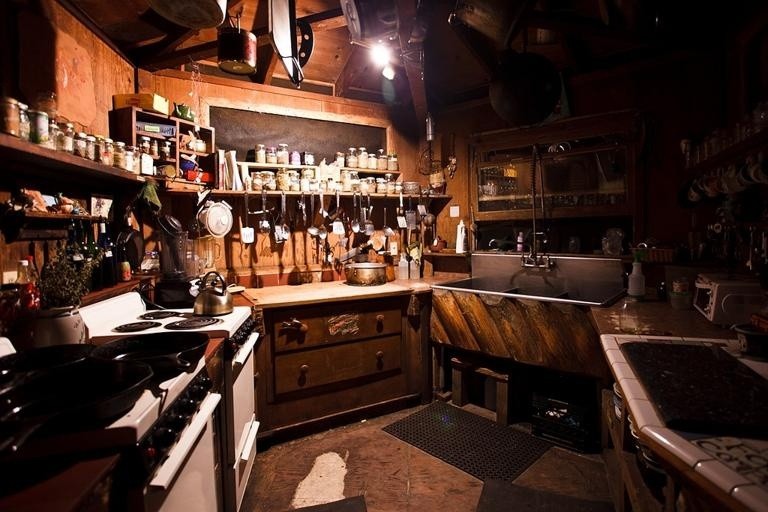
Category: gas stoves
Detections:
[
  {"x1": 89, "y1": 308, "x2": 260, "y2": 365},
  {"x1": 107, "y1": 352, "x2": 228, "y2": 489}
]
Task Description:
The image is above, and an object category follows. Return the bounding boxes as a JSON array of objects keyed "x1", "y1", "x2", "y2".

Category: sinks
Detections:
[
  {"x1": 431, "y1": 275, "x2": 516, "y2": 295},
  {"x1": 502, "y1": 286, "x2": 567, "y2": 302}
]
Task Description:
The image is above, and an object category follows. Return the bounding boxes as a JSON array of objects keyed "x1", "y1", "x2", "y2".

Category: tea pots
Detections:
[{"x1": 171, "y1": 101, "x2": 194, "y2": 122}]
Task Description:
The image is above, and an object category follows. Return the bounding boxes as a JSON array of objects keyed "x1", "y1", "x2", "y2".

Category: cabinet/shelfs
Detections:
[
  {"x1": 108, "y1": 107, "x2": 215, "y2": 193},
  {"x1": 216, "y1": 159, "x2": 455, "y2": 199},
  {"x1": 244, "y1": 279, "x2": 421, "y2": 446},
  {"x1": 468, "y1": 110, "x2": 644, "y2": 258},
  {"x1": 0, "y1": 133, "x2": 152, "y2": 321}
]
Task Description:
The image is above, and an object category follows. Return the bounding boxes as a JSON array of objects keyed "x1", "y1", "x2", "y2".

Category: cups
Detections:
[
  {"x1": 193, "y1": 236, "x2": 221, "y2": 268},
  {"x1": 156, "y1": 231, "x2": 189, "y2": 277},
  {"x1": 656, "y1": 281, "x2": 666, "y2": 300},
  {"x1": 535, "y1": 27, "x2": 555, "y2": 45},
  {"x1": 605, "y1": 228, "x2": 624, "y2": 256},
  {"x1": 687, "y1": 152, "x2": 768, "y2": 203},
  {"x1": 567, "y1": 236, "x2": 580, "y2": 253},
  {"x1": 403, "y1": 181, "x2": 420, "y2": 194}
]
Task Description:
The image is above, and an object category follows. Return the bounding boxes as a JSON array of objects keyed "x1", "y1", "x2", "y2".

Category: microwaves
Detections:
[{"x1": 691, "y1": 273, "x2": 766, "y2": 325}]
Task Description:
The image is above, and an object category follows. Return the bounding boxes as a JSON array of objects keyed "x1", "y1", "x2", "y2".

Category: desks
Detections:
[{"x1": 592, "y1": 294, "x2": 768, "y2": 511}]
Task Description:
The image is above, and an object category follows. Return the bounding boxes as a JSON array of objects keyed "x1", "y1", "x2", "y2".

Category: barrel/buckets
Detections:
[{"x1": 556, "y1": 290, "x2": 624, "y2": 305}]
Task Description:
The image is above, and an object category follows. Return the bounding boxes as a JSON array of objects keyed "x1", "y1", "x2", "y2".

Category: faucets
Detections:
[{"x1": 525, "y1": 247, "x2": 540, "y2": 267}]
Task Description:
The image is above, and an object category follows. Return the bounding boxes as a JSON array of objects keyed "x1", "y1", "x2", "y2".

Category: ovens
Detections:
[
  {"x1": 226, "y1": 333, "x2": 263, "y2": 512},
  {"x1": 144, "y1": 391, "x2": 229, "y2": 512}
]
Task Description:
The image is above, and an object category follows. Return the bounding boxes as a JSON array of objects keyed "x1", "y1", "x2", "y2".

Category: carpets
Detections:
[
  {"x1": 474, "y1": 479, "x2": 616, "y2": 512},
  {"x1": 382, "y1": 401, "x2": 552, "y2": 486},
  {"x1": 290, "y1": 494, "x2": 368, "y2": 512}
]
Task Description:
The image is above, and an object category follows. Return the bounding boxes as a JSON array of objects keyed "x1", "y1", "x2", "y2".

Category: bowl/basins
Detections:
[{"x1": 734, "y1": 323, "x2": 768, "y2": 361}]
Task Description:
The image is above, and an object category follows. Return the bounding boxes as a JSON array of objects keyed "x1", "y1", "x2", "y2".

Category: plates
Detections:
[{"x1": 611, "y1": 381, "x2": 664, "y2": 472}]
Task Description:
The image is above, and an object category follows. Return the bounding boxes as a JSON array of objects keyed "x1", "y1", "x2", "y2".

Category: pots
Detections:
[
  {"x1": 342, "y1": 261, "x2": 387, "y2": 287},
  {"x1": 1, "y1": 328, "x2": 210, "y2": 462},
  {"x1": 192, "y1": 271, "x2": 234, "y2": 316},
  {"x1": 431, "y1": 239, "x2": 447, "y2": 252}
]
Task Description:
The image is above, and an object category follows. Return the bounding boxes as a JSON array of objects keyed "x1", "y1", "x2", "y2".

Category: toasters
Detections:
[{"x1": 155, "y1": 281, "x2": 194, "y2": 309}]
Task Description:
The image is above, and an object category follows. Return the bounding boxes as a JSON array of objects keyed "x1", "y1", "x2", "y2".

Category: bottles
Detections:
[
  {"x1": 627, "y1": 248, "x2": 646, "y2": 296},
  {"x1": 33, "y1": 92, "x2": 58, "y2": 120},
  {"x1": 429, "y1": 159, "x2": 444, "y2": 185},
  {"x1": 254, "y1": 139, "x2": 399, "y2": 172},
  {"x1": 65, "y1": 214, "x2": 138, "y2": 284},
  {"x1": 516, "y1": 231, "x2": 524, "y2": 252},
  {"x1": 702, "y1": 121, "x2": 746, "y2": 156},
  {"x1": 0, "y1": 100, "x2": 172, "y2": 174},
  {"x1": 14, "y1": 254, "x2": 41, "y2": 313},
  {"x1": 251, "y1": 169, "x2": 404, "y2": 195},
  {"x1": 398, "y1": 253, "x2": 420, "y2": 280}
]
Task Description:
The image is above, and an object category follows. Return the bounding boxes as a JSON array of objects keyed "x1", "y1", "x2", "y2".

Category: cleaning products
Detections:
[{"x1": 627, "y1": 249, "x2": 650, "y2": 298}]
